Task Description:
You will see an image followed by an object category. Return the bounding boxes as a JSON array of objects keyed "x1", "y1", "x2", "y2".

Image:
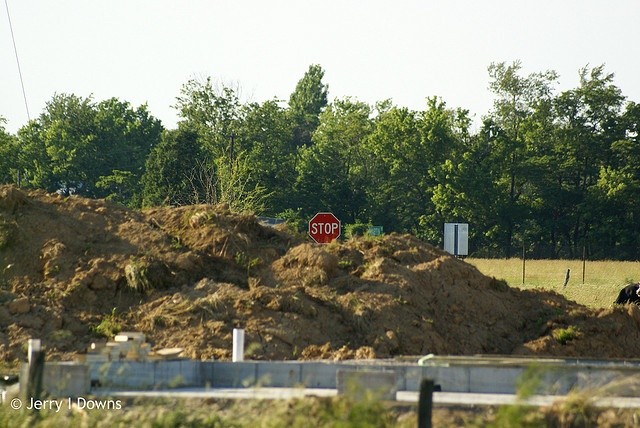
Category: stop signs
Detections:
[{"x1": 309, "y1": 213, "x2": 340, "y2": 244}]
